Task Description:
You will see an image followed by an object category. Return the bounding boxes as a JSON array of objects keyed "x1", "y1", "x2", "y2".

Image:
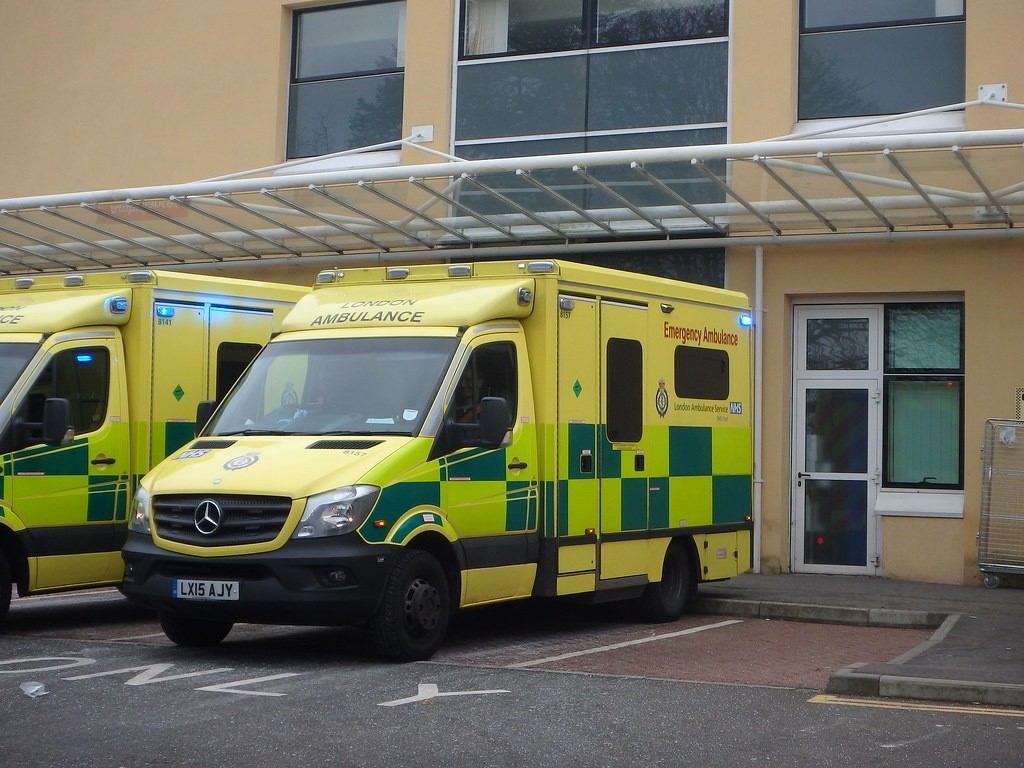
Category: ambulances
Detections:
[
  {"x1": 0, "y1": 269, "x2": 312, "y2": 626},
  {"x1": 115, "y1": 260, "x2": 754, "y2": 664}
]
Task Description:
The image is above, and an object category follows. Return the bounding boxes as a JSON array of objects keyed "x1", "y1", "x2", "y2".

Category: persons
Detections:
[{"x1": 455, "y1": 362, "x2": 496, "y2": 423}]
